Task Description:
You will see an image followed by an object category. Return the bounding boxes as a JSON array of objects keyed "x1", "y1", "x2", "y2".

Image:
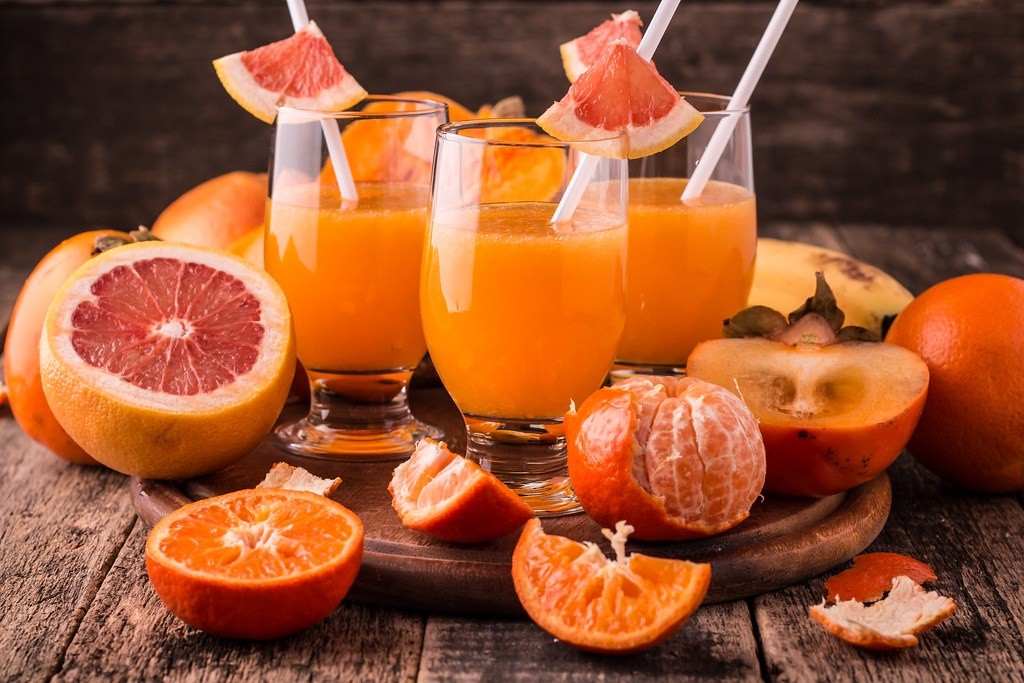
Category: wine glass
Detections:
[
  {"x1": 263, "y1": 95, "x2": 453, "y2": 462},
  {"x1": 418, "y1": 120, "x2": 627, "y2": 513},
  {"x1": 563, "y1": 93, "x2": 759, "y2": 385}
]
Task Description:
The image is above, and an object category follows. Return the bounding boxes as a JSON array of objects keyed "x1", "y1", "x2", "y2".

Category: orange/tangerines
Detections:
[{"x1": 1, "y1": 7, "x2": 1023, "y2": 652}]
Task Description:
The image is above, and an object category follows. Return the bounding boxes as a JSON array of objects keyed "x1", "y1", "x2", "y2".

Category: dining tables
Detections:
[{"x1": 1, "y1": 208, "x2": 1023, "y2": 682}]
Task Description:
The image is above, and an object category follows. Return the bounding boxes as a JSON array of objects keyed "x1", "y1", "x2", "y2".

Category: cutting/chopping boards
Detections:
[{"x1": 130, "y1": 352, "x2": 894, "y2": 614}]
